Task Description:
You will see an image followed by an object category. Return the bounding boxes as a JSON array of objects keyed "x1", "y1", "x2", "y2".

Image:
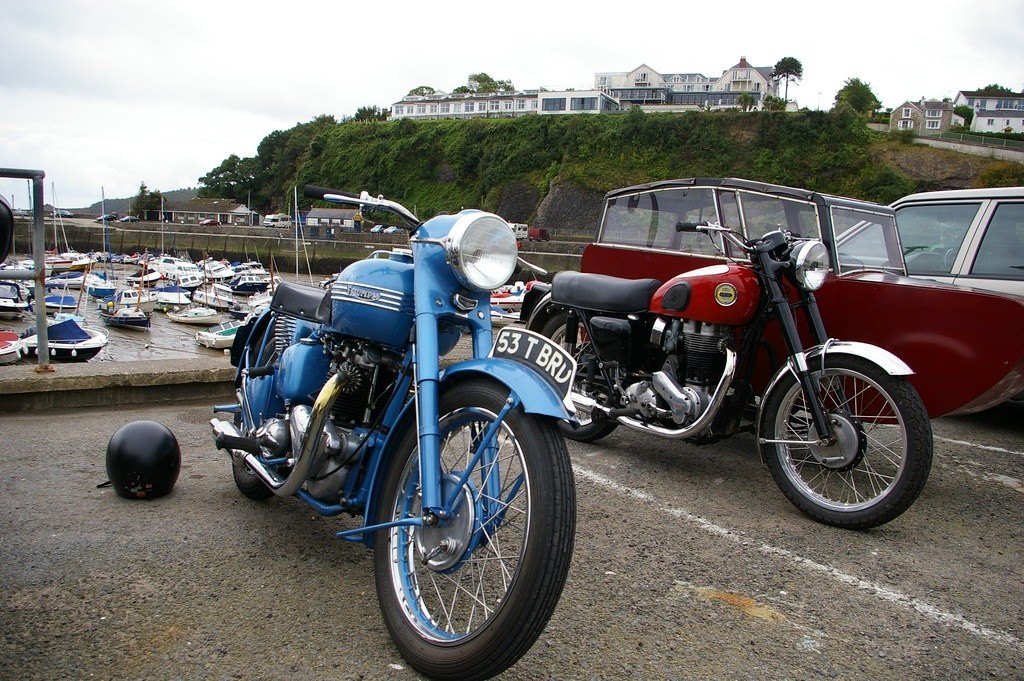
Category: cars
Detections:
[
  {"x1": 14, "y1": 207, "x2": 29, "y2": 217},
  {"x1": 807, "y1": 186, "x2": 1024, "y2": 321},
  {"x1": 120, "y1": 216, "x2": 140, "y2": 223},
  {"x1": 57, "y1": 210, "x2": 74, "y2": 218},
  {"x1": 370, "y1": 224, "x2": 386, "y2": 234},
  {"x1": 199, "y1": 218, "x2": 221, "y2": 227},
  {"x1": 97, "y1": 215, "x2": 117, "y2": 222},
  {"x1": 384, "y1": 226, "x2": 405, "y2": 235}
]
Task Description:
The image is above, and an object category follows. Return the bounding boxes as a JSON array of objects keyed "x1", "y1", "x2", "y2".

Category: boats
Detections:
[{"x1": 492, "y1": 281, "x2": 530, "y2": 325}]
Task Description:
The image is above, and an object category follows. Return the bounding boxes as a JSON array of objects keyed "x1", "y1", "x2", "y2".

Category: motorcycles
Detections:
[
  {"x1": 520, "y1": 221, "x2": 936, "y2": 533},
  {"x1": 211, "y1": 184, "x2": 584, "y2": 681}
]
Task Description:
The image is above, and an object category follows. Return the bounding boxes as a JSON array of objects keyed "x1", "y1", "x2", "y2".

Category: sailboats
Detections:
[{"x1": 0, "y1": 180, "x2": 289, "y2": 365}]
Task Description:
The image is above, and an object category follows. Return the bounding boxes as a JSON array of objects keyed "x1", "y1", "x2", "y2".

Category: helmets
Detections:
[{"x1": 106, "y1": 421, "x2": 182, "y2": 501}]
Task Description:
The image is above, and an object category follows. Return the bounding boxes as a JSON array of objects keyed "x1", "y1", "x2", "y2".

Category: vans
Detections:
[{"x1": 262, "y1": 215, "x2": 292, "y2": 229}]
